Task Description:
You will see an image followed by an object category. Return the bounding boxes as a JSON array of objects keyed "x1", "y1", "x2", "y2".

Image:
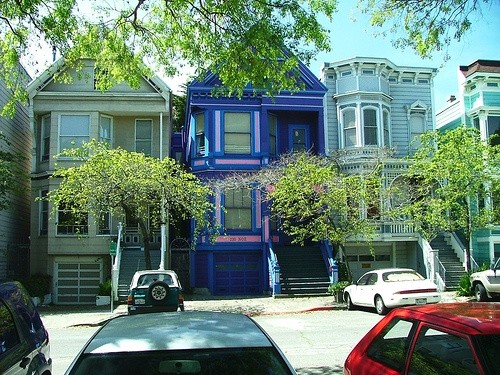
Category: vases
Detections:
[{"x1": 95, "y1": 278, "x2": 111, "y2": 306}]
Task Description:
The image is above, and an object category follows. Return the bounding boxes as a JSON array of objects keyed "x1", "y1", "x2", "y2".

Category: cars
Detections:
[
  {"x1": 63, "y1": 309, "x2": 298, "y2": 375},
  {"x1": 341, "y1": 268, "x2": 441, "y2": 315}
]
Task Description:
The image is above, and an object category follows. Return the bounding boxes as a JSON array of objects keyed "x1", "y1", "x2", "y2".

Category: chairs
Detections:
[{"x1": 163, "y1": 279, "x2": 172, "y2": 284}]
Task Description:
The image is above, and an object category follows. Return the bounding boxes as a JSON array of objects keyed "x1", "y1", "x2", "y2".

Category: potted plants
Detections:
[
  {"x1": 28, "y1": 271, "x2": 52, "y2": 306},
  {"x1": 327, "y1": 281, "x2": 350, "y2": 303}
]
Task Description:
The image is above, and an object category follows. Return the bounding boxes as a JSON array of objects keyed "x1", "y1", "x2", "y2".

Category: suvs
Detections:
[
  {"x1": 0, "y1": 280, "x2": 54, "y2": 375},
  {"x1": 469, "y1": 257, "x2": 500, "y2": 303},
  {"x1": 343, "y1": 300, "x2": 500, "y2": 375},
  {"x1": 127, "y1": 269, "x2": 185, "y2": 314}
]
